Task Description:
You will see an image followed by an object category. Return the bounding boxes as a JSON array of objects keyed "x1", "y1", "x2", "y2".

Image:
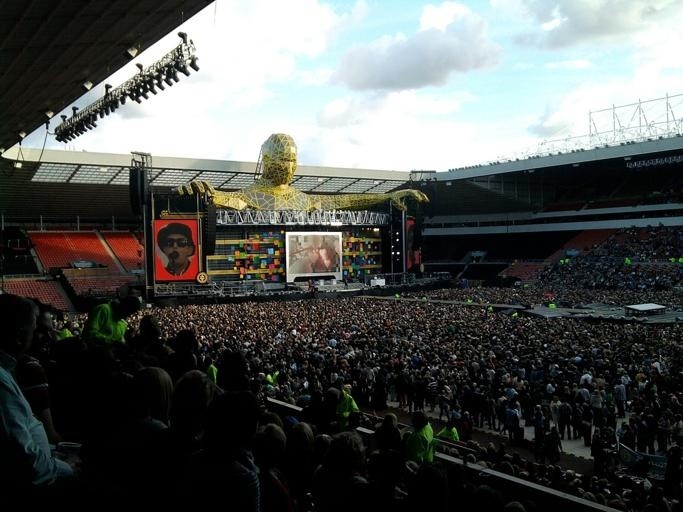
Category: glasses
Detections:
[{"x1": 162, "y1": 237, "x2": 188, "y2": 248}]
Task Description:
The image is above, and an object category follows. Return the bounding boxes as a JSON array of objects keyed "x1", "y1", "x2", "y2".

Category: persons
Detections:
[
  {"x1": 544, "y1": 218, "x2": 682, "y2": 269},
  {"x1": 156, "y1": 222, "x2": 196, "y2": 275},
  {"x1": 171, "y1": 131, "x2": 431, "y2": 215},
  {"x1": 310, "y1": 239, "x2": 340, "y2": 272},
  {"x1": 289, "y1": 236, "x2": 322, "y2": 274},
  {"x1": 0, "y1": 269, "x2": 682, "y2": 511}
]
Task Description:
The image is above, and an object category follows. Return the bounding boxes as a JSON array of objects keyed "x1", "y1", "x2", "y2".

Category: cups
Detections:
[{"x1": 57, "y1": 441, "x2": 80, "y2": 462}]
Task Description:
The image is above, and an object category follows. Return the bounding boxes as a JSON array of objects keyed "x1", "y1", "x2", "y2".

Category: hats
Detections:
[{"x1": 156, "y1": 222, "x2": 195, "y2": 258}]
